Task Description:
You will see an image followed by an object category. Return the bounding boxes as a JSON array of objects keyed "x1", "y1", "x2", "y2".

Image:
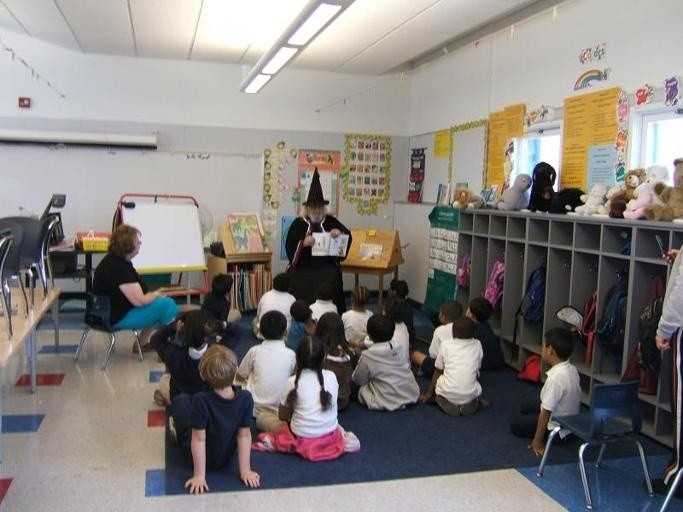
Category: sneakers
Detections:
[
  {"x1": 644, "y1": 478, "x2": 683, "y2": 500},
  {"x1": 460, "y1": 398, "x2": 481, "y2": 418},
  {"x1": 154, "y1": 390, "x2": 169, "y2": 406},
  {"x1": 168, "y1": 416, "x2": 178, "y2": 443},
  {"x1": 435, "y1": 395, "x2": 460, "y2": 418},
  {"x1": 133, "y1": 341, "x2": 164, "y2": 363}
]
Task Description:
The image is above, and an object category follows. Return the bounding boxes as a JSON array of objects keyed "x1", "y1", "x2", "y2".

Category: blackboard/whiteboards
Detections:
[
  {"x1": 0, "y1": 137, "x2": 265, "y2": 252},
  {"x1": 119, "y1": 192, "x2": 209, "y2": 276}
]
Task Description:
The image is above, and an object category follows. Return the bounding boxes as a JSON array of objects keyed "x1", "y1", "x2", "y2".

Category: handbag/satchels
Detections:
[{"x1": 621, "y1": 297, "x2": 665, "y2": 395}]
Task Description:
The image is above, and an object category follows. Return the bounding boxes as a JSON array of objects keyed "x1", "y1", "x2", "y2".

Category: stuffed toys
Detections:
[{"x1": 451, "y1": 158, "x2": 682, "y2": 229}]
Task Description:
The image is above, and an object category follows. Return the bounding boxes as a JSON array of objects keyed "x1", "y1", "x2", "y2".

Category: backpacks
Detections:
[
  {"x1": 583, "y1": 291, "x2": 597, "y2": 348},
  {"x1": 521, "y1": 268, "x2": 546, "y2": 325},
  {"x1": 457, "y1": 253, "x2": 471, "y2": 287},
  {"x1": 598, "y1": 270, "x2": 628, "y2": 355},
  {"x1": 484, "y1": 261, "x2": 505, "y2": 312}
]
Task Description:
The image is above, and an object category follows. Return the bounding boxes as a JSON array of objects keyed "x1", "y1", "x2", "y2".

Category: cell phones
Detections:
[{"x1": 655, "y1": 233, "x2": 675, "y2": 265}]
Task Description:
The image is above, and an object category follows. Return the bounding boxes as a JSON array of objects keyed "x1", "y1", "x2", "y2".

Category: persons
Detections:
[
  {"x1": 90, "y1": 225, "x2": 179, "y2": 354},
  {"x1": 281, "y1": 203, "x2": 347, "y2": 315},
  {"x1": 149, "y1": 268, "x2": 361, "y2": 495},
  {"x1": 643, "y1": 234, "x2": 683, "y2": 496},
  {"x1": 342, "y1": 278, "x2": 505, "y2": 418},
  {"x1": 510, "y1": 326, "x2": 583, "y2": 457}
]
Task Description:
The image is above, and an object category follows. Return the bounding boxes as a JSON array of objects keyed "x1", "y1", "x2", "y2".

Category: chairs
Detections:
[
  {"x1": 0, "y1": 208, "x2": 62, "y2": 343},
  {"x1": 72, "y1": 289, "x2": 146, "y2": 371},
  {"x1": 531, "y1": 382, "x2": 653, "y2": 509}
]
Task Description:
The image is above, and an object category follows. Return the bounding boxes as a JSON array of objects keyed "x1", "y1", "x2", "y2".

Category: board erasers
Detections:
[{"x1": 124, "y1": 202, "x2": 135, "y2": 208}]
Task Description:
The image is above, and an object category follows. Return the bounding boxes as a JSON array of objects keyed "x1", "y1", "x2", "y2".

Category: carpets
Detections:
[{"x1": 159, "y1": 292, "x2": 672, "y2": 498}]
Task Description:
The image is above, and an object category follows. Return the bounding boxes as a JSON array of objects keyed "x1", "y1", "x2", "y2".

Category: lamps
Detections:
[{"x1": 227, "y1": 1, "x2": 358, "y2": 98}]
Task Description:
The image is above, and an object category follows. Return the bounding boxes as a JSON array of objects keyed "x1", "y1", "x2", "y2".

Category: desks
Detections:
[
  {"x1": 338, "y1": 260, "x2": 402, "y2": 319},
  {"x1": 0, "y1": 281, "x2": 64, "y2": 403}
]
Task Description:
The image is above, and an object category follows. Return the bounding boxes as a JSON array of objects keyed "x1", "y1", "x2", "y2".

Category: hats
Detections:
[
  {"x1": 302, "y1": 166, "x2": 330, "y2": 206},
  {"x1": 556, "y1": 305, "x2": 583, "y2": 344}
]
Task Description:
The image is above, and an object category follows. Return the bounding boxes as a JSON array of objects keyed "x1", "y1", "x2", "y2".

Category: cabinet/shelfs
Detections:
[
  {"x1": 206, "y1": 250, "x2": 274, "y2": 320},
  {"x1": 448, "y1": 204, "x2": 683, "y2": 454}
]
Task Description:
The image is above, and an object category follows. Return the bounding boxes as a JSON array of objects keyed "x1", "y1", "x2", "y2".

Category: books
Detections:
[
  {"x1": 226, "y1": 262, "x2": 273, "y2": 313},
  {"x1": 309, "y1": 231, "x2": 348, "y2": 257}
]
